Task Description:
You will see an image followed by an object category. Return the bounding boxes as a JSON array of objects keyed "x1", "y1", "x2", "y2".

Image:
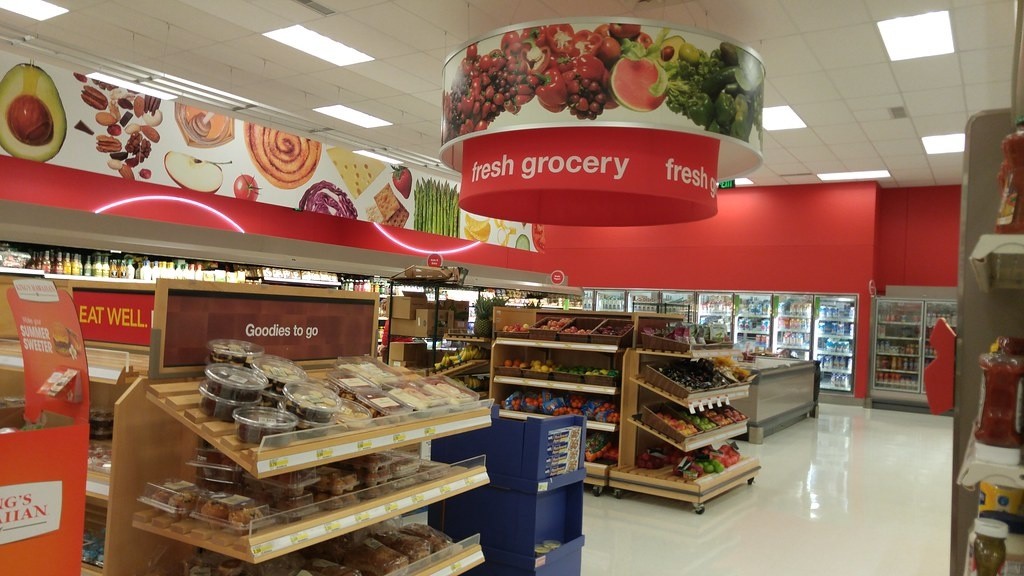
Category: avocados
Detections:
[{"x1": 0, "y1": 59, "x2": 67, "y2": 162}]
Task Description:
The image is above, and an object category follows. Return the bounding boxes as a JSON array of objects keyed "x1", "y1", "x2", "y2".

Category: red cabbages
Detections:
[{"x1": 299, "y1": 180, "x2": 358, "y2": 220}]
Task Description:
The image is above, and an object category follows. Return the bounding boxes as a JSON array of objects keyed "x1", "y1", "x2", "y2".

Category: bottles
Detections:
[
  {"x1": 26, "y1": 249, "x2": 203, "y2": 283},
  {"x1": 925, "y1": 343, "x2": 937, "y2": 356},
  {"x1": 874, "y1": 372, "x2": 919, "y2": 393},
  {"x1": 903, "y1": 325, "x2": 912, "y2": 337},
  {"x1": 602, "y1": 296, "x2": 625, "y2": 309},
  {"x1": 756, "y1": 335, "x2": 769, "y2": 344},
  {"x1": 926, "y1": 309, "x2": 956, "y2": 327},
  {"x1": 996, "y1": 110, "x2": 1024, "y2": 237},
  {"x1": 341, "y1": 276, "x2": 453, "y2": 302},
  {"x1": 738, "y1": 318, "x2": 752, "y2": 331},
  {"x1": 782, "y1": 333, "x2": 810, "y2": 345},
  {"x1": 877, "y1": 340, "x2": 920, "y2": 355},
  {"x1": 878, "y1": 309, "x2": 921, "y2": 323},
  {"x1": 973, "y1": 336, "x2": 1024, "y2": 446},
  {"x1": 962, "y1": 482, "x2": 1024, "y2": 575},
  {"x1": 779, "y1": 297, "x2": 812, "y2": 316},
  {"x1": 740, "y1": 300, "x2": 771, "y2": 315},
  {"x1": 378, "y1": 326, "x2": 385, "y2": 340},
  {"x1": 876, "y1": 355, "x2": 919, "y2": 371},
  {"x1": 776, "y1": 317, "x2": 811, "y2": 331}
]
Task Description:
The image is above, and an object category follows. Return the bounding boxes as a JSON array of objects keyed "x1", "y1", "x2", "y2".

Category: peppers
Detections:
[
  {"x1": 532, "y1": 71, "x2": 568, "y2": 107},
  {"x1": 666, "y1": 43, "x2": 755, "y2": 143},
  {"x1": 520, "y1": 40, "x2": 551, "y2": 76}
]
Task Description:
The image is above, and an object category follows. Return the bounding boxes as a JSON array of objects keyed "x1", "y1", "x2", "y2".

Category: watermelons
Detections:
[{"x1": 609, "y1": 57, "x2": 666, "y2": 111}]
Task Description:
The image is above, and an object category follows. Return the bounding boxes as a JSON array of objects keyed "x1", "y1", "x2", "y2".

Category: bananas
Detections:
[{"x1": 434, "y1": 346, "x2": 486, "y2": 389}]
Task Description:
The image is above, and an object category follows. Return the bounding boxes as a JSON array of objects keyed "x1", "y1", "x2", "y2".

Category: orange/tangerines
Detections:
[
  {"x1": 501, "y1": 394, "x2": 619, "y2": 461},
  {"x1": 556, "y1": 366, "x2": 594, "y2": 382}
]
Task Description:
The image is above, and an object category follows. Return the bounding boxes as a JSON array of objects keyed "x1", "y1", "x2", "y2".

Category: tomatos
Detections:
[
  {"x1": 444, "y1": 23, "x2": 652, "y2": 140},
  {"x1": 234, "y1": 174, "x2": 260, "y2": 201}
]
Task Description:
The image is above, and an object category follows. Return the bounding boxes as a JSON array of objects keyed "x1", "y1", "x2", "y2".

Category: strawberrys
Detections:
[{"x1": 390, "y1": 164, "x2": 412, "y2": 200}]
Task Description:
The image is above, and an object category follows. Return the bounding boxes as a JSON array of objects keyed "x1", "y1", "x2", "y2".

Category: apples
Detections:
[
  {"x1": 164, "y1": 151, "x2": 232, "y2": 193},
  {"x1": 640, "y1": 445, "x2": 739, "y2": 481},
  {"x1": 656, "y1": 404, "x2": 745, "y2": 437}
]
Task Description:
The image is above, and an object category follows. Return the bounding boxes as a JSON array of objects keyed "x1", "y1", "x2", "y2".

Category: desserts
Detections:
[{"x1": 201, "y1": 343, "x2": 474, "y2": 448}]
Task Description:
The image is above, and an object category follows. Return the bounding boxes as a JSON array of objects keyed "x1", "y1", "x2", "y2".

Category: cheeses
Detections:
[{"x1": 326, "y1": 145, "x2": 386, "y2": 199}]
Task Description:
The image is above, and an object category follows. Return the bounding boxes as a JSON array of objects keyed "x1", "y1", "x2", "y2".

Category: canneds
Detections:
[
  {"x1": 963, "y1": 518, "x2": 1009, "y2": 576},
  {"x1": 974, "y1": 353, "x2": 1024, "y2": 466}
]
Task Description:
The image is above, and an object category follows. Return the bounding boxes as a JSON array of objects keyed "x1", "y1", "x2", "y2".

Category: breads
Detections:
[{"x1": 149, "y1": 453, "x2": 455, "y2": 576}]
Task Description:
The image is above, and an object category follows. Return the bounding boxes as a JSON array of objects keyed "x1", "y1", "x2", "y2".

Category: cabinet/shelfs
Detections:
[
  {"x1": 949, "y1": 108, "x2": 1024, "y2": 576},
  {"x1": 0, "y1": 198, "x2": 762, "y2": 576}
]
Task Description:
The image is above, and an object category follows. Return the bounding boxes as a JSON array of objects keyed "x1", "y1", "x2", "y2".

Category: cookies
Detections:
[{"x1": 366, "y1": 184, "x2": 409, "y2": 228}]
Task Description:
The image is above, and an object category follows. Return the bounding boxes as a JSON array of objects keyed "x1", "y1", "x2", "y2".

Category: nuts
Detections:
[
  {"x1": 96, "y1": 135, "x2": 122, "y2": 152},
  {"x1": 80, "y1": 86, "x2": 107, "y2": 110}
]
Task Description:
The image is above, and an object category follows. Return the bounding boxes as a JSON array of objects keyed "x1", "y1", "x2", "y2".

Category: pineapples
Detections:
[{"x1": 474, "y1": 294, "x2": 506, "y2": 336}]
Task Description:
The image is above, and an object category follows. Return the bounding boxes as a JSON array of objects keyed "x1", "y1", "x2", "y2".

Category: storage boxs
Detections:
[
  {"x1": 414, "y1": 309, "x2": 449, "y2": 338},
  {"x1": 388, "y1": 340, "x2": 428, "y2": 368},
  {"x1": 385, "y1": 291, "x2": 427, "y2": 320},
  {"x1": 390, "y1": 318, "x2": 415, "y2": 337}
]
